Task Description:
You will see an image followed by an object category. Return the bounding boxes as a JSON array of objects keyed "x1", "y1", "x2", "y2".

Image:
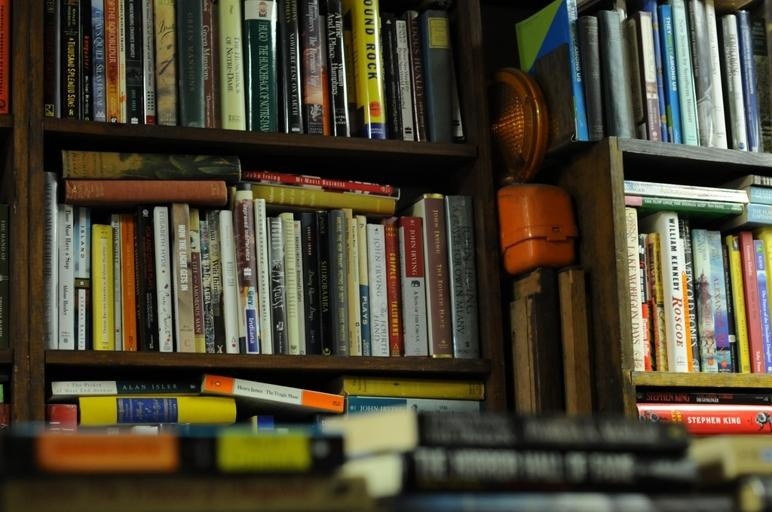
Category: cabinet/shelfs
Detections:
[
  {"x1": 564, "y1": 136, "x2": 771, "y2": 434},
  {"x1": 31, "y1": 0, "x2": 513, "y2": 417}
]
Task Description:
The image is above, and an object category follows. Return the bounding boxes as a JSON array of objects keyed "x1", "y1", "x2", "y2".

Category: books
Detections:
[
  {"x1": 0, "y1": 0, "x2": 13, "y2": 353},
  {"x1": 43, "y1": 1, "x2": 468, "y2": 145},
  {"x1": 623, "y1": 175, "x2": 772, "y2": 375},
  {"x1": 45, "y1": 151, "x2": 481, "y2": 360},
  {"x1": 514, "y1": 2, "x2": 772, "y2": 159},
  {"x1": 0, "y1": 364, "x2": 771, "y2": 512}
]
